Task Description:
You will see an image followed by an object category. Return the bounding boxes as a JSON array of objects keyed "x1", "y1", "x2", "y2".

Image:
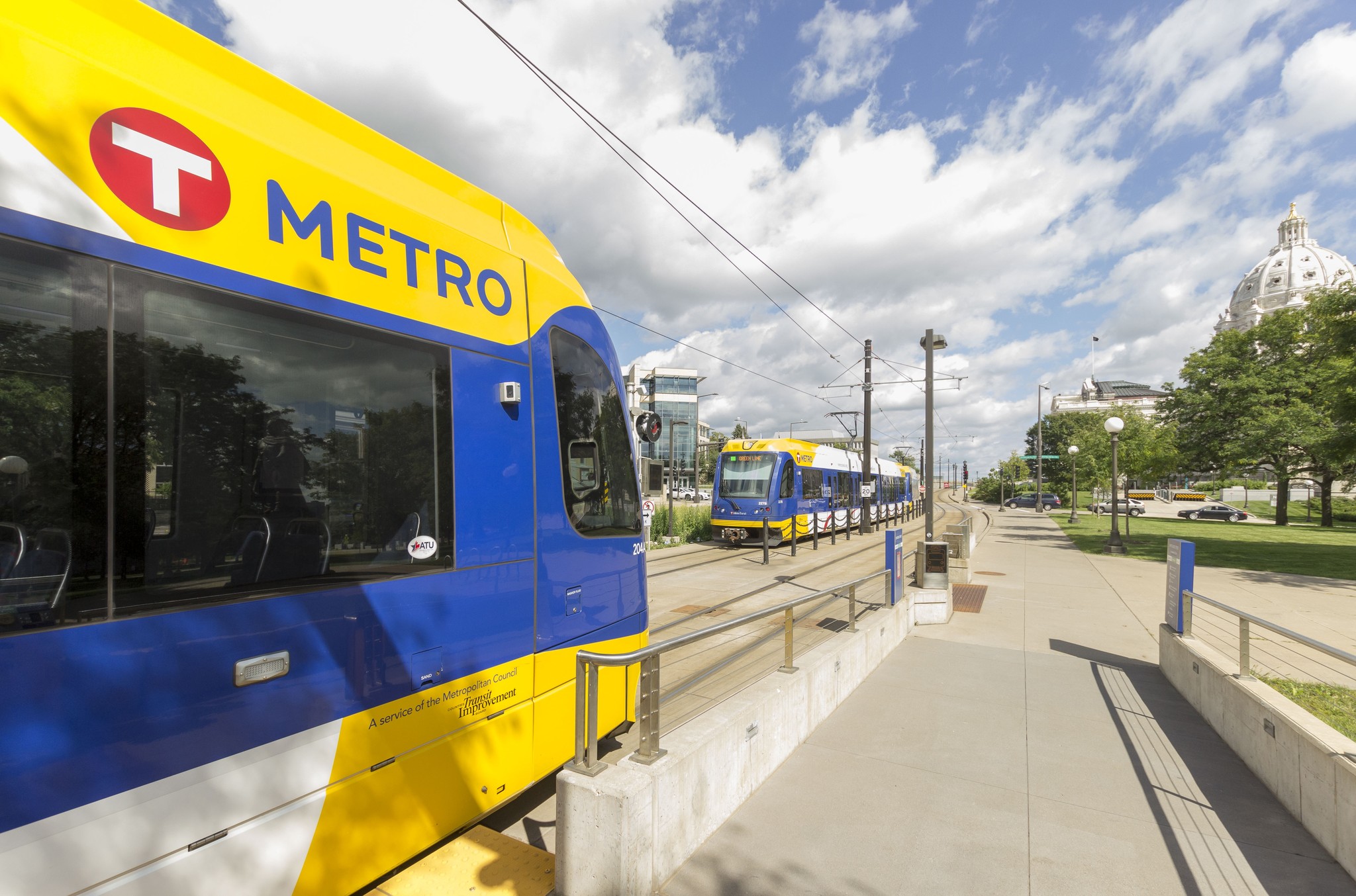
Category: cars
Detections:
[
  {"x1": 667, "y1": 487, "x2": 711, "y2": 501},
  {"x1": 1087, "y1": 498, "x2": 1248, "y2": 522},
  {"x1": 1003, "y1": 494, "x2": 1061, "y2": 511}
]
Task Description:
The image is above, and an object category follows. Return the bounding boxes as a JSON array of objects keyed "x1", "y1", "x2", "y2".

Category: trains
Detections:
[
  {"x1": 0, "y1": 0, "x2": 662, "y2": 896},
  {"x1": 710, "y1": 438, "x2": 918, "y2": 547}
]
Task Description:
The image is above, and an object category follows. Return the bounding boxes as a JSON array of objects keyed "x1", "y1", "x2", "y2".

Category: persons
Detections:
[{"x1": 0, "y1": 456, "x2": 46, "y2": 537}]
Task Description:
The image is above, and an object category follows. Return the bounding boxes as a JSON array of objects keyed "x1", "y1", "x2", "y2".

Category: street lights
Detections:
[
  {"x1": 734, "y1": 419, "x2": 808, "y2": 439},
  {"x1": 1035, "y1": 385, "x2": 1050, "y2": 513},
  {"x1": 1067, "y1": 417, "x2": 1128, "y2": 552},
  {"x1": 1243, "y1": 473, "x2": 1250, "y2": 508},
  {"x1": 661, "y1": 393, "x2": 719, "y2": 542},
  {"x1": 1123, "y1": 477, "x2": 1127, "y2": 498},
  {"x1": 893, "y1": 447, "x2": 915, "y2": 466},
  {"x1": 955, "y1": 463, "x2": 958, "y2": 491},
  {"x1": 952, "y1": 464, "x2": 956, "y2": 496},
  {"x1": 962, "y1": 460, "x2": 967, "y2": 501},
  {"x1": 976, "y1": 471, "x2": 978, "y2": 481},
  {"x1": 1211, "y1": 465, "x2": 1217, "y2": 495}
]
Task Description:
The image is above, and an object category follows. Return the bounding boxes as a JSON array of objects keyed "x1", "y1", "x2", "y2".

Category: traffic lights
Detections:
[
  {"x1": 640, "y1": 459, "x2": 664, "y2": 496},
  {"x1": 963, "y1": 465, "x2": 968, "y2": 479},
  {"x1": 673, "y1": 459, "x2": 686, "y2": 480},
  {"x1": 998, "y1": 465, "x2": 1020, "y2": 480},
  {"x1": 1029, "y1": 460, "x2": 1037, "y2": 479}
]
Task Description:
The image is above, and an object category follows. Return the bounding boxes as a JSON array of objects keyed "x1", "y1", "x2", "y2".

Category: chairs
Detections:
[
  {"x1": 144, "y1": 508, "x2": 432, "y2": 591},
  {"x1": 0, "y1": 522, "x2": 73, "y2": 614}
]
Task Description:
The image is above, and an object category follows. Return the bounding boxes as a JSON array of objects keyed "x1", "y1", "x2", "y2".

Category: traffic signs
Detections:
[{"x1": 642, "y1": 509, "x2": 652, "y2": 527}]
